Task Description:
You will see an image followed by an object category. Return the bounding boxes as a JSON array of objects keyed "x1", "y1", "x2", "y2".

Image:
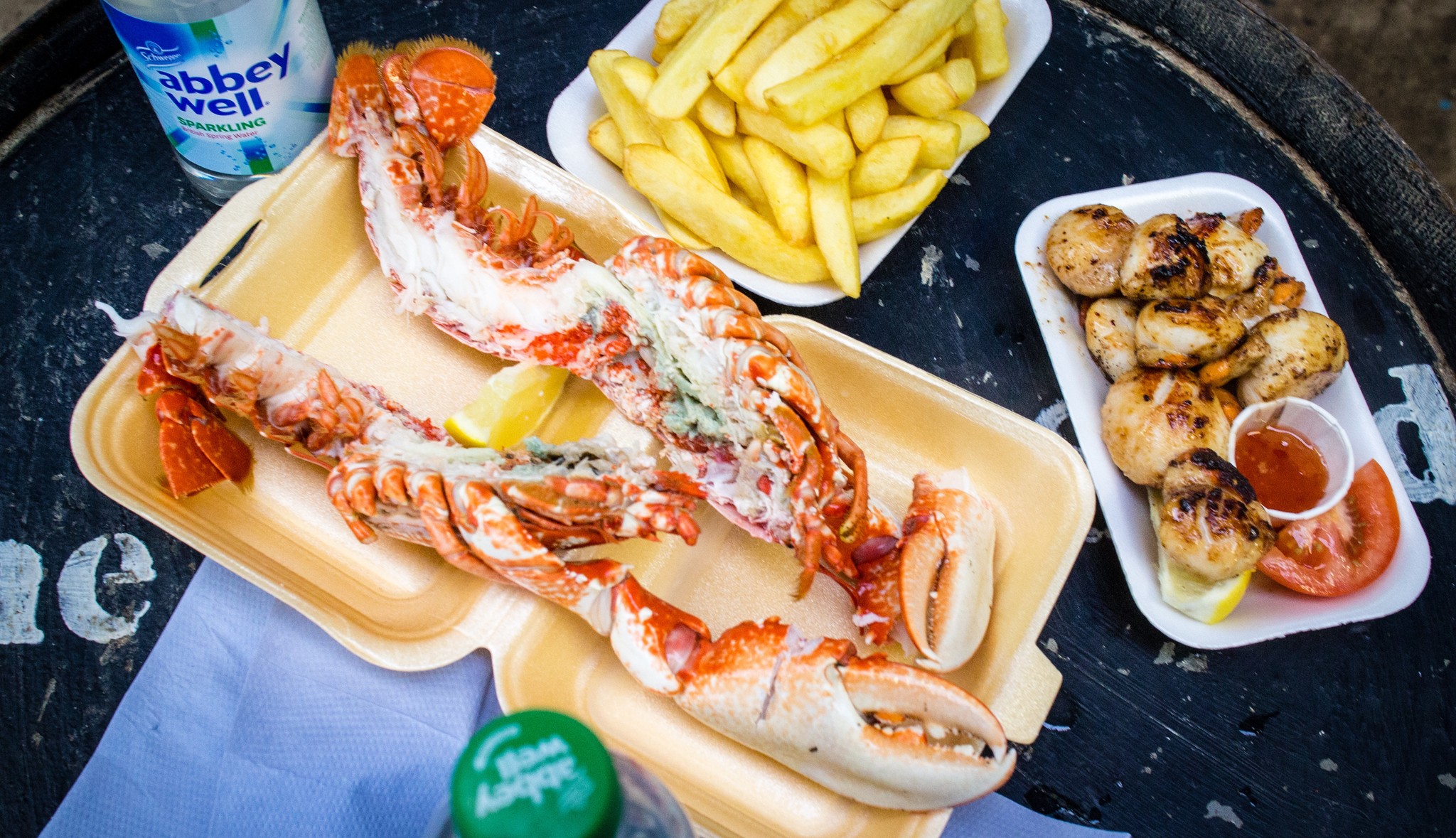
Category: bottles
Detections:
[
  {"x1": 448, "y1": 712, "x2": 697, "y2": 838},
  {"x1": 104, "y1": 0, "x2": 341, "y2": 206}
]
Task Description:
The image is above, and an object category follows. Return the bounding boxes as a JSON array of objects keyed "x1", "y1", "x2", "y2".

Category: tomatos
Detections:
[{"x1": 1256, "y1": 459, "x2": 1401, "y2": 598}]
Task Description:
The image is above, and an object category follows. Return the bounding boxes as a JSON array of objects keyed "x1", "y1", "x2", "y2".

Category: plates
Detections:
[
  {"x1": 1014, "y1": 171, "x2": 1431, "y2": 652},
  {"x1": 545, "y1": 0, "x2": 1054, "y2": 308}
]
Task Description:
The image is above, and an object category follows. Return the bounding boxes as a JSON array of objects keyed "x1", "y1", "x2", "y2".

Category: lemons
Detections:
[
  {"x1": 442, "y1": 358, "x2": 570, "y2": 454},
  {"x1": 1144, "y1": 484, "x2": 1250, "y2": 626}
]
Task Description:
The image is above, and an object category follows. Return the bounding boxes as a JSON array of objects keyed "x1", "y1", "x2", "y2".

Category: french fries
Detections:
[{"x1": 587, "y1": 0, "x2": 1012, "y2": 301}]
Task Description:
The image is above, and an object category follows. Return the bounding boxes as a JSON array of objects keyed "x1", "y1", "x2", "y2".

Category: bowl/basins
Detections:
[{"x1": 1229, "y1": 398, "x2": 1357, "y2": 528}]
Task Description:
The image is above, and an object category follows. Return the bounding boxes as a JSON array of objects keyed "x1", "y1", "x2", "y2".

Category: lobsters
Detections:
[{"x1": 72, "y1": 30, "x2": 1019, "y2": 813}]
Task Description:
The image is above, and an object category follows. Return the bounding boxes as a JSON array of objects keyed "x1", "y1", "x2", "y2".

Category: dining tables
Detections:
[{"x1": 5, "y1": 2, "x2": 1456, "y2": 838}]
